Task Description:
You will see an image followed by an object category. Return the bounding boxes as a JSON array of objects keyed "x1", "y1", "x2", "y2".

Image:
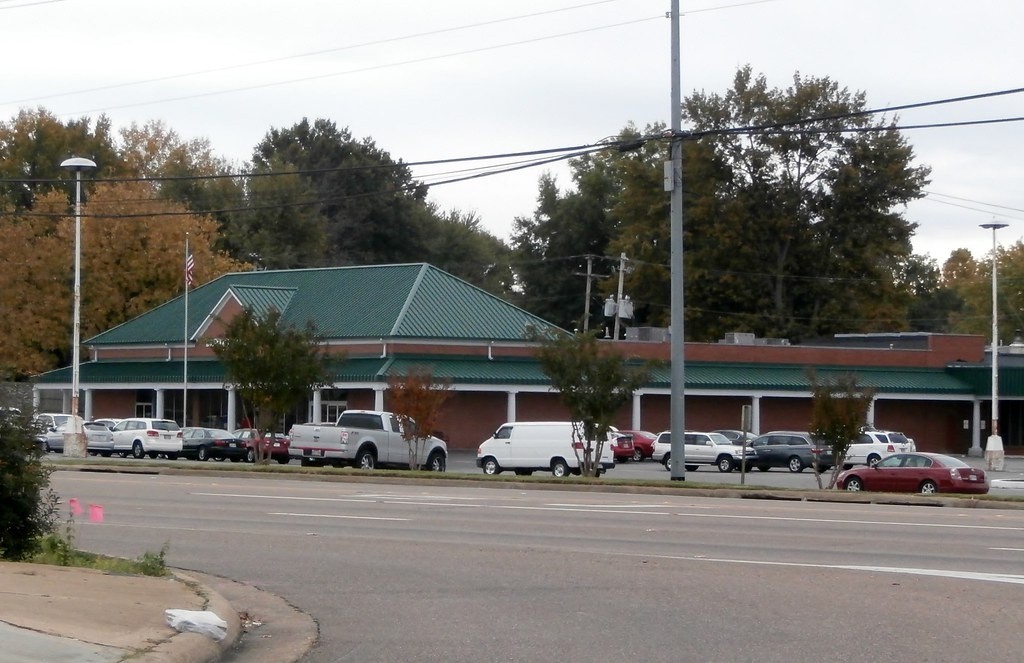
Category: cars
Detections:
[
  {"x1": 93, "y1": 418, "x2": 125, "y2": 430},
  {"x1": 158, "y1": 426, "x2": 247, "y2": 463},
  {"x1": 835, "y1": 451, "x2": 989, "y2": 494},
  {"x1": 609, "y1": 425, "x2": 659, "y2": 463},
  {"x1": 213, "y1": 428, "x2": 291, "y2": 463},
  {"x1": 35, "y1": 421, "x2": 115, "y2": 457},
  {"x1": 704, "y1": 429, "x2": 760, "y2": 446}
]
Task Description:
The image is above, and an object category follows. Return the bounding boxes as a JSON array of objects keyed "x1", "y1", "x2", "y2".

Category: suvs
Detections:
[
  {"x1": 652, "y1": 431, "x2": 759, "y2": 473},
  {"x1": 736, "y1": 430, "x2": 836, "y2": 473},
  {"x1": 811, "y1": 428, "x2": 916, "y2": 473}
]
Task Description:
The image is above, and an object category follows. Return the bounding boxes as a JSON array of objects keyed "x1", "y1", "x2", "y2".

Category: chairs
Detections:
[{"x1": 905, "y1": 458, "x2": 917, "y2": 467}]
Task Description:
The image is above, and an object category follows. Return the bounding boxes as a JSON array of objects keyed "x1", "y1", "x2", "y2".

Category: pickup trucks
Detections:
[{"x1": 287, "y1": 409, "x2": 448, "y2": 473}]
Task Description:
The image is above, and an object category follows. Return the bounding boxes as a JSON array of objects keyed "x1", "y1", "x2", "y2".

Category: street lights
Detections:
[
  {"x1": 978, "y1": 220, "x2": 1009, "y2": 435},
  {"x1": 60, "y1": 157, "x2": 97, "y2": 433}
]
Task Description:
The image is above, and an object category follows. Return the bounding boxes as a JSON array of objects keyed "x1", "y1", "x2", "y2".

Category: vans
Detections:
[
  {"x1": 475, "y1": 420, "x2": 615, "y2": 477},
  {"x1": 31, "y1": 412, "x2": 83, "y2": 435},
  {"x1": 111, "y1": 417, "x2": 183, "y2": 461}
]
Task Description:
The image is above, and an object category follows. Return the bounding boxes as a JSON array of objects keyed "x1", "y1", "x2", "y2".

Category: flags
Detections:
[{"x1": 184, "y1": 238, "x2": 194, "y2": 285}]
